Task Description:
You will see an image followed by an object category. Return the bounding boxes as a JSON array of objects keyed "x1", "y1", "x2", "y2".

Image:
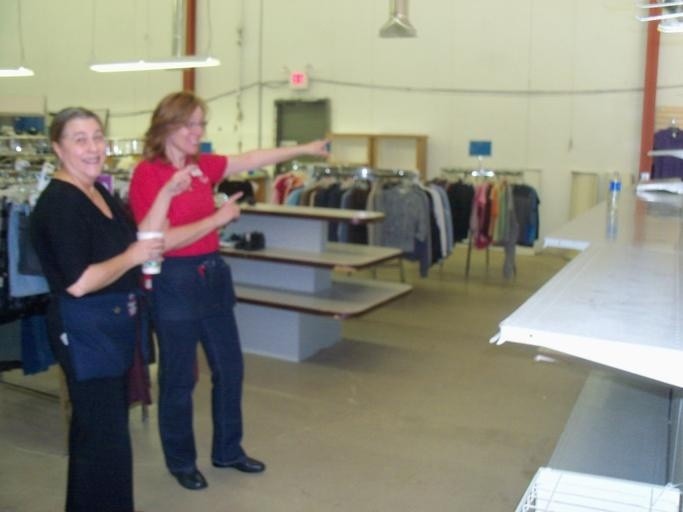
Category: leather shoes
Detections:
[
  {"x1": 166, "y1": 463, "x2": 208, "y2": 490},
  {"x1": 210, "y1": 454, "x2": 266, "y2": 474}
]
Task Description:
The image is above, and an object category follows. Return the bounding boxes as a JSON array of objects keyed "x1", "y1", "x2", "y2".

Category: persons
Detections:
[
  {"x1": 126, "y1": 90, "x2": 332, "y2": 490},
  {"x1": 29, "y1": 105, "x2": 193, "y2": 512}
]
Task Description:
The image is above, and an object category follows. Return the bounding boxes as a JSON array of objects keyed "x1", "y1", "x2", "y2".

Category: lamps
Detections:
[
  {"x1": 0, "y1": 66, "x2": 35, "y2": 79},
  {"x1": 90, "y1": 51, "x2": 221, "y2": 71}
]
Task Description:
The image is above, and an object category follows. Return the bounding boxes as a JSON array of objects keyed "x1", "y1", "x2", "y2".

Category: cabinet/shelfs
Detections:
[
  {"x1": 488, "y1": 1, "x2": 683, "y2": 512},
  {"x1": 220, "y1": 206, "x2": 412, "y2": 364}
]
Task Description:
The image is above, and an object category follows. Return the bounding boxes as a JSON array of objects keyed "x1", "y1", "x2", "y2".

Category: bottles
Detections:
[
  {"x1": 604, "y1": 207, "x2": 620, "y2": 242},
  {"x1": 608, "y1": 169, "x2": 621, "y2": 209}
]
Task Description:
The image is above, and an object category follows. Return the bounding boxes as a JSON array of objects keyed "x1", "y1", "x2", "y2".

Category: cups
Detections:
[{"x1": 136, "y1": 231, "x2": 166, "y2": 276}]
